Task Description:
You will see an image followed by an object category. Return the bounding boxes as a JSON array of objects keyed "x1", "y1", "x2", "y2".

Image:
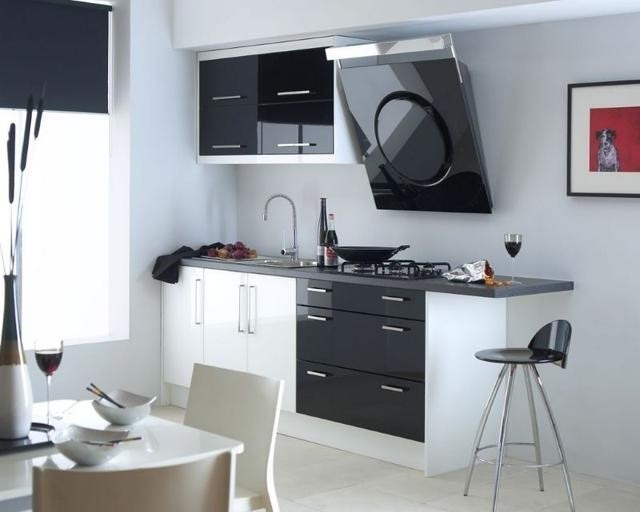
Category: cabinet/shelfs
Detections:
[
  {"x1": 204, "y1": 267, "x2": 296, "y2": 413},
  {"x1": 162, "y1": 265, "x2": 204, "y2": 390},
  {"x1": 199, "y1": 46, "x2": 335, "y2": 155},
  {"x1": 296, "y1": 276, "x2": 425, "y2": 446}
]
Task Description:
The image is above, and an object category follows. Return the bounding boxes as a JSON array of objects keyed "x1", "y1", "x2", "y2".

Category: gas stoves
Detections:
[{"x1": 322, "y1": 259, "x2": 452, "y2": 281}]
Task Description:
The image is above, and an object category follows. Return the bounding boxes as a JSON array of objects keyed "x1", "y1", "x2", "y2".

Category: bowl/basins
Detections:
[
  {"x1": 50, "y1": 424, "x2": 131, "y2": 467},
  {"x1": 91, "y1": 386, "x2": 159, "y2": 427}
]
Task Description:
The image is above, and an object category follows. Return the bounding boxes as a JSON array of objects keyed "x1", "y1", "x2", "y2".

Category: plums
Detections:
[{"x1": 208, "y1": 242, "x2": 257, "y2": 258}]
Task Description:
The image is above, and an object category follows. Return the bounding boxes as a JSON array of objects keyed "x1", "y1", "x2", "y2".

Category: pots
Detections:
[{"x1": 319, "y1": 242, "x2": 412, "y2": 267}]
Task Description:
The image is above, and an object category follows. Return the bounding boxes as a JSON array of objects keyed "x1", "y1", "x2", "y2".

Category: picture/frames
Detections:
[{"x1": 567, "y1": 79, "x2": 640, "y2": 197}]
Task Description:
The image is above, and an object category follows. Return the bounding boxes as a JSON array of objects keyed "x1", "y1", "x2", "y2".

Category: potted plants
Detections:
[{"x1": 0, "y1": 82, "x2": 48, "y2": 440}]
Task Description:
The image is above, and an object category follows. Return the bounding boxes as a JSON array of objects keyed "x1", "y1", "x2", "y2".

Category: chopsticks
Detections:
[
  {"x1": 86, "y1": 382, "x2": 126, "y2": 408},
  {"x1": 80, "y1": 436, "x2": 142, "y2": 448}
]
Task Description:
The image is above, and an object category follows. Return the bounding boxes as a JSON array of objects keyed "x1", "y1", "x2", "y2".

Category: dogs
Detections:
[{"x1": 596, "y1": 128, "x2": 618, "y2": 172}]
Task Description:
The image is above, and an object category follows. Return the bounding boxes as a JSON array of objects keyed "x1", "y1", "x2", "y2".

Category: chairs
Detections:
[
  {"x1": 32, "y1": 448, "x2": 233, "y2": 511},
  {"x1": 180, "y1": 362, "x2": 286, "y2": 512},
  {"x1": 462, "y1": 319, "x2": 576, "y2": 512}
]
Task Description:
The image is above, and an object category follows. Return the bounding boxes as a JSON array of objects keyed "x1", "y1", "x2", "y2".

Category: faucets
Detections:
[{"x1": 263, "y1": 192, "x2": 299, "y2": 261}]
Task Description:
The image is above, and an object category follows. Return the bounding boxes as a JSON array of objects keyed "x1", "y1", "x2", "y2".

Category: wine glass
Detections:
[
  {"x1": 33, "y1": 338, "x2": 64, "y2": 427},
  {"x1": 502, "y1": 232, "x2": 524, "y2": 286}
]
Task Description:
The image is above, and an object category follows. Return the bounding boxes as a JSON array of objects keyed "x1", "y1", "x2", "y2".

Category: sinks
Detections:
[{"x1": 241, "y1": 257, "x2": 317, "y2": 269}]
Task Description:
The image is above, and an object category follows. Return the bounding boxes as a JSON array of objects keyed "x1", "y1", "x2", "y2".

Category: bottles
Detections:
[
  {"x1": 322, "y1": 213, "x2": 339, "y2": 268},
  {"x1": 316, "y1": 197, "x2": 329, "y2": 269}
]
